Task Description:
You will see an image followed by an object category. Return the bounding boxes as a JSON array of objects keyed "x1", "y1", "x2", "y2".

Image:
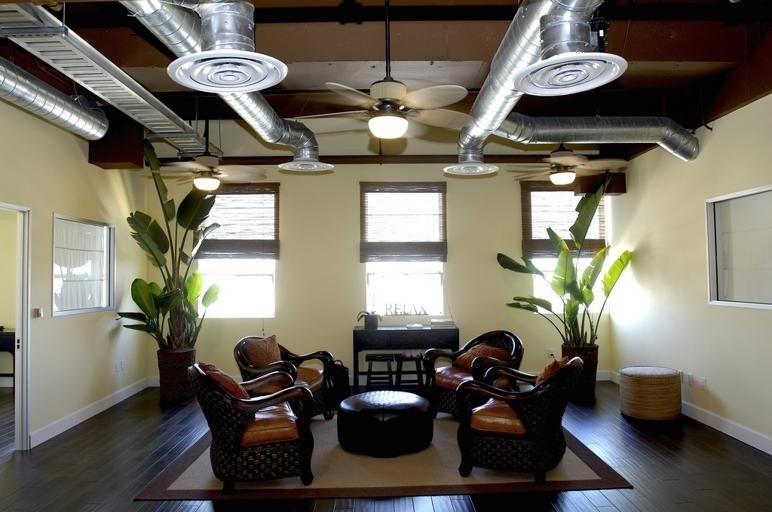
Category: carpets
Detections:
[{"x1": 131, "y1": 412, "x2": 634, "y2": 501}]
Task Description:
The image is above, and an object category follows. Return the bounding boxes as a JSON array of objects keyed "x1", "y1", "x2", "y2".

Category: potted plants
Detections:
[
  {"x1": 497, "y1": 176, "x2": 633, "y2": 408},
  {"x1": 115, "y1": 137, "x2": 220, "y2": 409},
  {"x1": 356, "y1": 310, "x2": 383, "y2": 330}
]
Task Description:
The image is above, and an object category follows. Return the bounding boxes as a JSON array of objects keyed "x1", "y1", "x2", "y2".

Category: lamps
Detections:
[
  {"x1": 192, "y1": 171, "x2": 220, "y2": 192},
  {"x1": 548, "y1": 166, "x2": 577, "y2": 185},
  {"x1": 366, "y1": 99, "x2": 408, "y2": 139}
]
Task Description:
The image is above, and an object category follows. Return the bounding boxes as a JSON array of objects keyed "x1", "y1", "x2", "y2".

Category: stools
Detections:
[
  {"x1": 618, "y1": 366, "x2": 681, "y2": 422},
  {"x1": 364, "y1": 353, "x2": 395, "y2": 385},
  {"x1": 394, "y1": 352, "x2": 424, "y2": 386},
  {"x1": 336, "y1": 390, "x2": 434, "y2": 458}
]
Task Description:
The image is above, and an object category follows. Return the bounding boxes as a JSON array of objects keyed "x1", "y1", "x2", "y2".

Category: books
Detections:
[{"x1": 430, "y1": 318, "x2": 456, "y2": 329}]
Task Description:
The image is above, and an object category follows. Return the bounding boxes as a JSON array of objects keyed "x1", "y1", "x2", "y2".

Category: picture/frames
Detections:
[{"x1": 705, "y1": 183, "x2": 772, "y2": 309}]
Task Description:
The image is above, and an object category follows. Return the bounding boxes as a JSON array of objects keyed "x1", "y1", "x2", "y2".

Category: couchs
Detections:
[
  {"x1": 186, "y1": 360, "x2": 317, "y2": 496},
  {"x1": 422, "y1": 329, "x2": 523, "y2": 417},
  {"x1": 233, "y1": 335, "x2": 349, "y2": 421},
  {"x1": 456, "y1": 357, "x2": 585, "y2": 486}
]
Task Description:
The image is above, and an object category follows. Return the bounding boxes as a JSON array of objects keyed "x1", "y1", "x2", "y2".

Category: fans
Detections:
[
  {"x1": 293, "y1": 22, "x2": 473, "y2": 130},
  {"x1": 505, "y1": 142, "x2": 630, "y2": 182},
  {"x1": 138, "y1": 117, "x2": 267, "y2": 183}
]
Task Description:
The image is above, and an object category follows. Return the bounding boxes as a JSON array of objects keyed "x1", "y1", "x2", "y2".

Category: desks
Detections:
[{"x1": 352, "y1": 323, "x2": 461, "y2": 392}]
